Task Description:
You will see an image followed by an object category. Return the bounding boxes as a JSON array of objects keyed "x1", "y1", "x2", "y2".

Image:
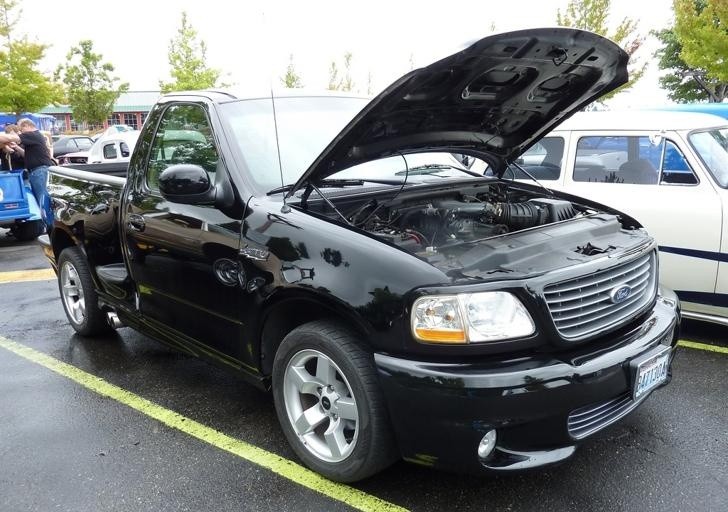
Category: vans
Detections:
[{"x1": 464, "y1": 111, "x2": 727, "y2": 328}]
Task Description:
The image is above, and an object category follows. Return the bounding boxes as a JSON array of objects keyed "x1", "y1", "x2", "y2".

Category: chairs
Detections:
[
  {"x1": 576, "y1": 166, "x2": 612, "y2": 182},
  {"x1": 618, "y1": 159, "x2": 659, "y2": 184}
]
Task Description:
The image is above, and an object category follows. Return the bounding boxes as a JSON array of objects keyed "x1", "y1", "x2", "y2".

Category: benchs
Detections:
[{"x1": 486, "y1": 166, "x2": 560, "y2": 180}]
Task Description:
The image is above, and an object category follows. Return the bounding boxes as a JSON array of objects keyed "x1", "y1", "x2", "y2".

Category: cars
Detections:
[{"x1": 53, "y1": 127, "x2": 207, "y2": 165}]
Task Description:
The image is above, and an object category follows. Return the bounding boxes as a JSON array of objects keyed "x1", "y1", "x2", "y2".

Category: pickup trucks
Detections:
[{"x1": 36, "y1": 26, "x2": 679, "y2": 482}]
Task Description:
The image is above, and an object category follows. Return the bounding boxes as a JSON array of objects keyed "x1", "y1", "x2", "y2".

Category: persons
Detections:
[
  {"x1": 0, "y1": 118, "x2": 55, "y2": 230},
  {"x1": 0, "y1": 124, "x2": 26, "y2": 169}
]
